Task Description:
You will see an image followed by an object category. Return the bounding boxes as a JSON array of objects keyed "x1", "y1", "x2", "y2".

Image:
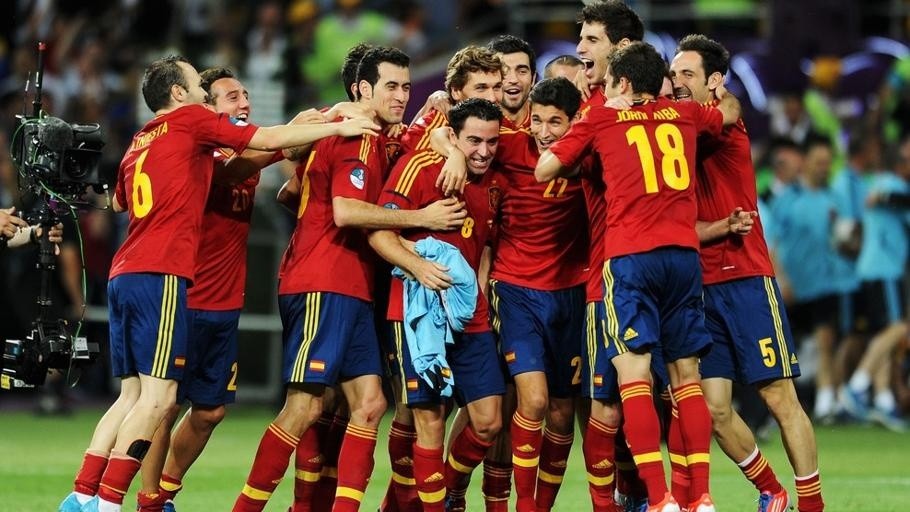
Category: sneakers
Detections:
[
  {"x1": 682, "y1": 493, "x2": 716, "y2": 512},
  {"x1": 639, "y1": 491, "x2": 681, "y2": 512},
  {"x1": 758, "y1": 488, "x2": 794, "y2": 512}
]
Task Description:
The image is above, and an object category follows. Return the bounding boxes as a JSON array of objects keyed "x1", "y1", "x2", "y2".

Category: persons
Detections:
[{"x1": 1, "y1": 1, "x2": 910, "y2": 512}]
